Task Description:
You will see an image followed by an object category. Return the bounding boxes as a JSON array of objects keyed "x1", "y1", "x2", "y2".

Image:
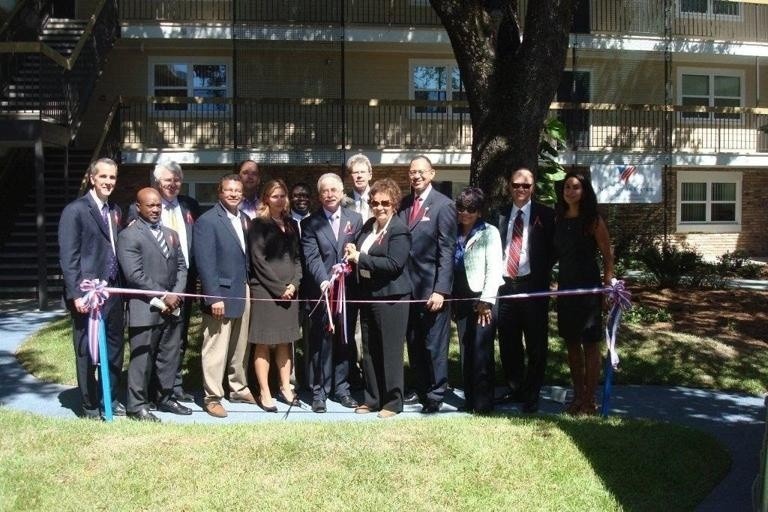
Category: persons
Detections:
[
  {"x1": 499, "y1": 168, "x2": 560, "y2": 414},
  {"x1": 127, "y1": 160, "x2": 195, "y2": 403},
  {"x1": 345, "y1": 153, "x2": 374, "y2": 225},
  {"x1": 192, "y1": 173, "x2": 257, "y2": 418},
  {"x1": 116, "y1": 188, "x2": 192, "y2": 423},
  {"x1": 300, "y1": 172, "x2": 363, "y2": 412},
  {"x1": 288, "y1": 182, "x2": 312, "y2": 238},
  {"x1": 396, "y1": 155, "x2": 458, "y2": 414},
  {"x1": 236, "y1": 160, "x2": 262, "y2": 221},
  {"x1": 246, "y1": 178, "x2": 303, "y2": 414},
  {"x1": 450, "y1": 186, "x2": 506, "y2": 418},
  {"x1": 555, "y1": 169, "x2": 613, "y2": 415},
  {"x1": 56, "y1": 157, "x2": 127, "y2": 422},
  {"x1": 344, "y1": 177, "x2": 414, "y2": 418}
]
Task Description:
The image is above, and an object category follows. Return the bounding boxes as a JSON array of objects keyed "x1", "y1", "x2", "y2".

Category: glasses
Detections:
[
  {"x1": 457, "y1": 205, "x2": 476, "y2": 213},
  {"x1": 511, "y1": 183, "x2": 531, "y2": 189}
]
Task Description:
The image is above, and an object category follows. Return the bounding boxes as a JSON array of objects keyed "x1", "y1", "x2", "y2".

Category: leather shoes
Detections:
[
  {"x1": 81, "y1": 388, "x2": 193, "y2": 424},
  {"x1": 457, "y1": 392, "x2": 540, "y2": 415},
  {"x1": 202, "y1": 389, "x2": 442, "y2": 416}
]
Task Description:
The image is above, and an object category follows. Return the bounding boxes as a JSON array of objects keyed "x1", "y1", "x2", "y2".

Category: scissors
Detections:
[{"x1": 308, "y1": 254, "x2": 348, "y2": 317}]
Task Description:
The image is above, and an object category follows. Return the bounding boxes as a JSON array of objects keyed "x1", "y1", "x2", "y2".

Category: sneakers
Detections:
[{"x1": 568, "y1": 394, "x2": 598, "y2": 414}]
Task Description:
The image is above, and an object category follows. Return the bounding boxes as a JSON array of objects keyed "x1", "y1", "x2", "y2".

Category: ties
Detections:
[
  {"x1": 360, "y1": 196, "x2": 367, "y2": 225},
  {"x1": 167, "y1": 202, "x2": 177, "y2": 230},
  {"x1": 507, "y1": 210, "x2": 523, "y2": 279},
  {"x1": 155, "y1": 225, "x2": 169, "y2": 259},
  {"x1": 409, "y1": 196, "x2": 419, "y2": 225},
  {"x1": 329, "y1": 214, "x2": 338, "y2": 240},
  {"x1": 101, "y1": 204, "x2": 118, "y2": 282}
]
